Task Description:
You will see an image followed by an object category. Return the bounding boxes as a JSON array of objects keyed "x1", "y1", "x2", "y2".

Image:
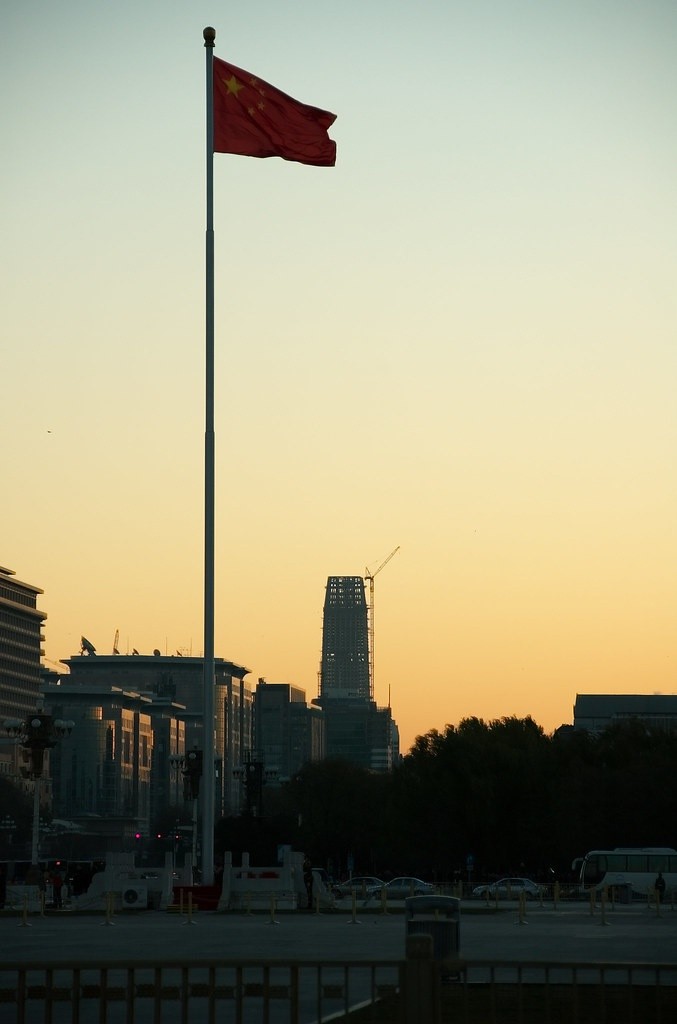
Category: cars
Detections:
[
  {"x1": 473, "y1": 878, "x2": 548, "y2": 899},
  {"x1": 331, "y1": 875, "x2": 387, "y2": 900},
  {"x1": 370, "y1": 876, "x2": 435, "y2": 902}
]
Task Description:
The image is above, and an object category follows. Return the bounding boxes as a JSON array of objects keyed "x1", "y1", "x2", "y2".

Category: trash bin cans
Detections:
[
  {"x1": 618, "y1": 883, "x2": 633, "y2": 905},
  {"x1": 403, "y1": 892, "x2": 462, "y2": 978}
]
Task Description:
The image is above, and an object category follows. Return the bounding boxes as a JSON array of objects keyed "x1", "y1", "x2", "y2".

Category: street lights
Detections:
[{"x1": 2, "y1": 698, "x2": 76, "y2": 887}]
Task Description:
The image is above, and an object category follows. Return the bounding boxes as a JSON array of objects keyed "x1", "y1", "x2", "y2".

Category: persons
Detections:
[
  {"x1": 35, "y1": 863, "x2": 99, "y2": 914},
  {"x1": 299, "y1": 853, "x2": 315, "y2": 915},
  {"x1": 655, "y1": 872, "x2": 666, "y2": 905}
]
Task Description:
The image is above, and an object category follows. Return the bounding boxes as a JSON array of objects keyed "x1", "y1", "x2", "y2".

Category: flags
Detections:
[{"x1": 212, "y1": 56, "x2": 337, "y2": 166}]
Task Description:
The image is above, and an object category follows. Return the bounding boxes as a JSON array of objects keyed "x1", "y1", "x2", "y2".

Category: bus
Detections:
[{"x1": 572, "y1": 847, "x2": 677, "y2": 903}]
